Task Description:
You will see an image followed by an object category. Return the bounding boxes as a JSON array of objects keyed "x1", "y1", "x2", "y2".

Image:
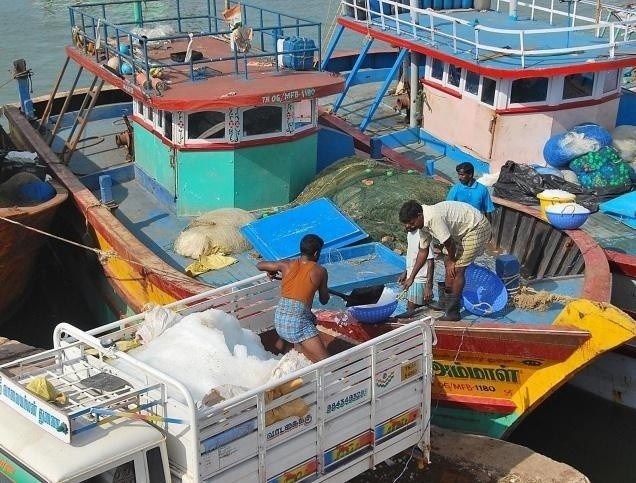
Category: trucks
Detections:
[{"x1": 0, "y1": 262, "x2": 439, "y2": 483}]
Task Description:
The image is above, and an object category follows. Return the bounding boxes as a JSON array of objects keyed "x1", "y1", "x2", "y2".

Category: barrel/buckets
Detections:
[
  {"x1": 0, "y1": 169, "x2": 56, "y2": 208},
  {"x1": 277, "y1": 36, "x2": 315, "y2": 69},
  {"x1": 344, "y1": 0, "x2": 491, "y2": 20},
  {"x1": 537, "y1": 193, "x2": 577, "y2": 220}
]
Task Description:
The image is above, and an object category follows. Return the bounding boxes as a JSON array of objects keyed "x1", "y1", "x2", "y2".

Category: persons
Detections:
[
  {"x1": 398, "y1": 224, "x2": 434, "y2": 310},
  {"x1": 445, "y1": 162, "x2": 495, "y2": 224},
  {"x1": 400, "y1": 200, "x2": 492, "y2": 322},
  {"x1": 256, "y1": 233, "x2": 331, "y2": 361}
]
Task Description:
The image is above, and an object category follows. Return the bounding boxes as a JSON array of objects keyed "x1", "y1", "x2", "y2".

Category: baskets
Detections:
[
  {"x1": 544, "y1": 203, "x2": 592, "y2": 230},
  {"x1": 537, "y1": 189, "x2": 576, "y2": 214},
  {"x1": 14, "y1": 182, "x2": 56, "y2": 203},
  {"x1": 463, "y1": 261, "x2": 508, "y2": 316},
  {"x1": 348, "y1": 299, "x2": 397, "y2": 325}
]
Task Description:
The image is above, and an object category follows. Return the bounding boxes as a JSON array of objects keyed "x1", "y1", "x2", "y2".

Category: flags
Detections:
[{"x1": 222, "y1": 4, "x2": 242, "y2": 30}]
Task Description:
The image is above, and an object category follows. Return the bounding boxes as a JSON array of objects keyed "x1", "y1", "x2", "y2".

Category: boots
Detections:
[
  {"x1": 430, "y1": 290, "x2": 448, "y2": 311},
  {"x1": 436, "y1": 289, "x2": 462, "y2": 321}
]
